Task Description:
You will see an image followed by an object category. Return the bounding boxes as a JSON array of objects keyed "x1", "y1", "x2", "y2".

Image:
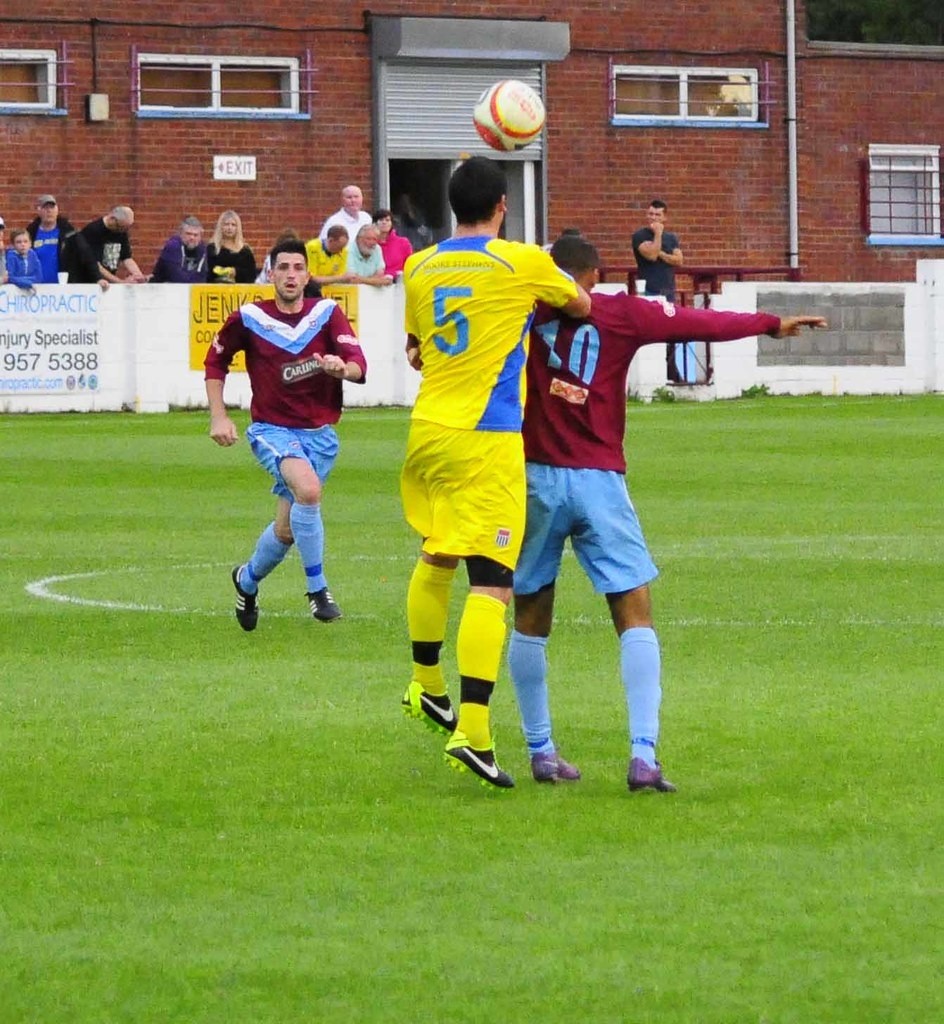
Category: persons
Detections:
[
  {"x1": 149, "y1": 209, "x2": 257, "y2": 282},
  {"x1": 0, "y1": 195, "x2": 146, "y2": 294},
  {"x1": 508, "y1": 226, "x2": 828, "y2": 793},
  {"x1": 632, "y1": 200, "x2": 684, "y2": 383},
  {"x1": 255, "y1": 184, "x2": 412, "y2": 287},
  {"x1": 203, "y1": 241, "x2": 367, "y2": 631},
  {"x1": 400, "y1": 157, "x2": 592, "y2": 794}
]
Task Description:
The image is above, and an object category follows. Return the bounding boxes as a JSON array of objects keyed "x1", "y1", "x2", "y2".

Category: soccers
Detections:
[{"x1": 473, "y1": 79, "x2": 546, "y2": 153}]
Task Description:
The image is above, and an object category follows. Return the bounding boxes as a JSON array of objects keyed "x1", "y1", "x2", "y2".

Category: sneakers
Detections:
[
  {"x1": 400, "y1": 680, "x2": 459, "y2": 736},
  {"x1": 304, "y1": 586, "x2": 341, "y2": 623},
  {"x1": 231, "y1": 563, "x2": 260, "y2": 631},
  {"x1": 628, "y1": 757, "x2": 676, "y2": 792},
  {"x1": 530, "y1": 747, "x2": 581, "y2": 781},
  {"x1": 443, "y1": 730, "x2": 514, "y2": 788}
]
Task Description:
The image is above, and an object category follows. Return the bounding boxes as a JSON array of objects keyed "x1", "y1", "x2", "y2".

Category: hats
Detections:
[{"x1": 38, "y1": 194, "x2": 57, "y2": 209}]
